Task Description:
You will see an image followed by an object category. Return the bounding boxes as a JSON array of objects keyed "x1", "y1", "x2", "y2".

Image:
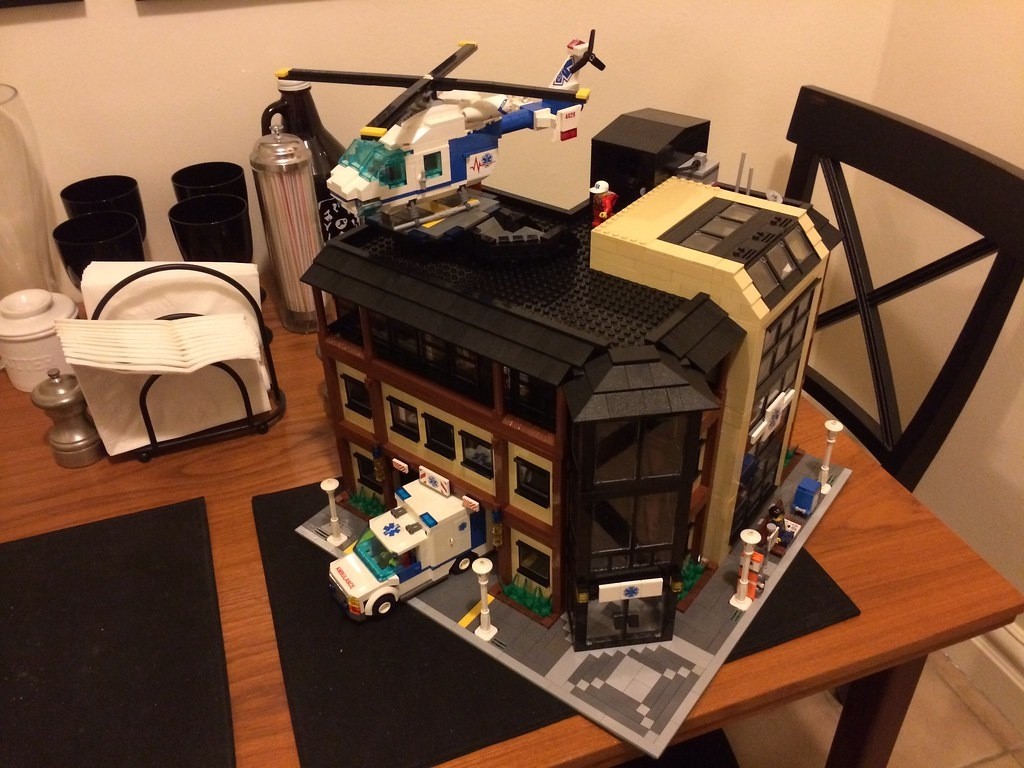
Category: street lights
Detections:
[
  {"x1": 819, "y1": 419, "x2": 843, "y2": 494},
  {"x1": 729, "y1": 527, "x2": 763, "y2": 612},
  {"x1": 322, "y1": 478, "x2": 347, "y2": 550},
  {"x1": 472, "y1": 557, "x2": 498, "y2": 642}
]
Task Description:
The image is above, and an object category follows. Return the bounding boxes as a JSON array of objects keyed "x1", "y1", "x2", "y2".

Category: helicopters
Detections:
[{"x1": 274, "y1": 29, "x2": 607, "y2": 224}]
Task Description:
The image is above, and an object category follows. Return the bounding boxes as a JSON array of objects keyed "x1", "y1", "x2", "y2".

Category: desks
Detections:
[{"x1": 0, "y1": 301, "x2": 1024, "y2": 768}]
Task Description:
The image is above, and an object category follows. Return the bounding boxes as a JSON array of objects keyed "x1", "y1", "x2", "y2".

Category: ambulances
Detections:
[{"x1": 328, "y1": 477, "x2": 498, "y2": 623}]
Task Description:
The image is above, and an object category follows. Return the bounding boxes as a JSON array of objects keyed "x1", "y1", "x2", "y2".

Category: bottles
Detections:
[{"x1": 260, "y1": 66, "x2": 359, "y2": 245}]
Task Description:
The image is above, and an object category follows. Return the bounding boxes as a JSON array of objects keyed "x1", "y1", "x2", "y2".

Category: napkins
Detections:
[{"x1": 54, "y1": 259, "x2": 274, "y2": 458}]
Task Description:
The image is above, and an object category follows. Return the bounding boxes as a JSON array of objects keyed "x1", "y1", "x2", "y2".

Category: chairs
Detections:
[{"x1": 782, "y1": 84, "x2": 1024, "y2": 492}]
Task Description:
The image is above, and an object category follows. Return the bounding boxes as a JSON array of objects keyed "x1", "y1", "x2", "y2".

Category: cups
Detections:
[
  {"x1": 52, "y1": 171, "x2": 149, "y2": 291},
  {"x1": 166, "y1": 161, "x2": 274, "y2": 349},
  {"x1": 0, "y1": 83, "x2": 61, "y2": 310}
]
